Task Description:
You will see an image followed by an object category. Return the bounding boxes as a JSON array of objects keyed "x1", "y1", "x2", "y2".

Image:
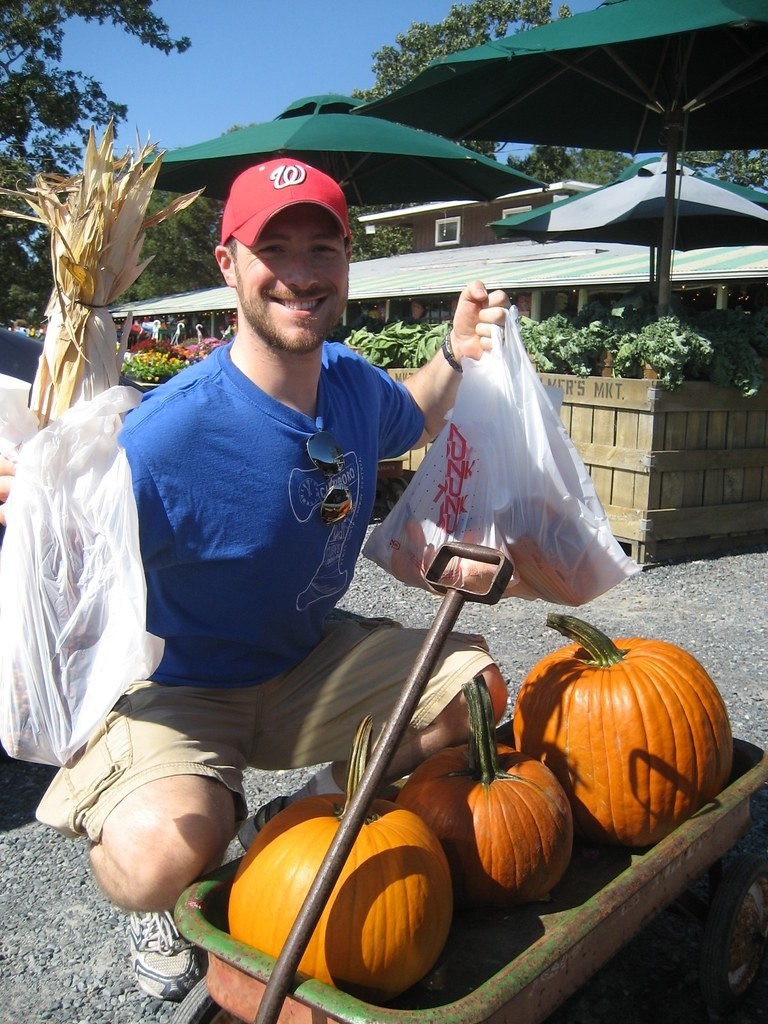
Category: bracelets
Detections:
[{"x1": 441, "y1": 333, "x2": 462, "y2": 372}]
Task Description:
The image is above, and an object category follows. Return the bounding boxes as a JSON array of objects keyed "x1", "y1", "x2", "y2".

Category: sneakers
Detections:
[
  {"x1": 238, "y1": 795, "x2": 285, "y2": 851},
  {"x1": 130, "y1": 910, "x2": 201, "y2": 999}
]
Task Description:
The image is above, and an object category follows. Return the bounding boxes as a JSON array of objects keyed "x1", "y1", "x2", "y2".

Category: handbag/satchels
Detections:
[
  {"x1": 363, "y1": 302, "x2": 643, "y2": 605},
  {"x1": 0, "y1": 384, "x2": 166, "y2": 766}
]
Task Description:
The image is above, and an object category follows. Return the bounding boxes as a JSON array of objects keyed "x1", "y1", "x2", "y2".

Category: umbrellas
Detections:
[
  {"x1": 120, "y1": 94, "x2": 549, "y2": 204},
  {"x1": 351, "y1": 0, "x2": 768, "y2": 322},
  {"x1": 487, "y1": 156, "x2": 768, "y2": 289}
]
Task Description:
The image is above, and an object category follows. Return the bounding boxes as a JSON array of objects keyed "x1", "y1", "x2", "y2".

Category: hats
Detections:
[{"x1": 220, "y1": 157, "x2": 353, "y2": 247}]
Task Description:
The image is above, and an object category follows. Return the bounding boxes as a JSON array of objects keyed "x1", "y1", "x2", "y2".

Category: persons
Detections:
[{"x1": 0, "y1": 158, "x2": 523, "y2": 998}]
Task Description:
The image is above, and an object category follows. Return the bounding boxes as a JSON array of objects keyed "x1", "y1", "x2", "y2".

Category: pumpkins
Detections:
[
  {"x1": 395, "y1": 675, "x2": 576, "y2": 907},
  {"x1": 227, "y1": 710, "x2": 455, "y2": 1005},
  {"x1": 510, "y1": 611, "x2": 732, "y2": 848}
]
